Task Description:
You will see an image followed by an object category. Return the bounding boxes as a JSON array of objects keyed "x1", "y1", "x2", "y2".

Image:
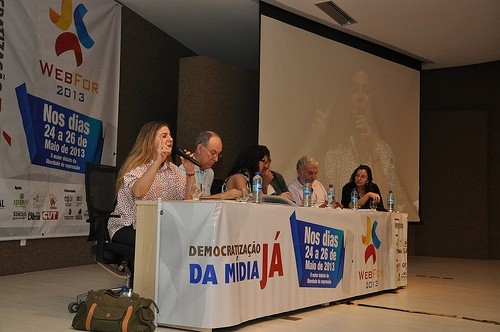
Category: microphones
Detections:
[{"x1": 176, "y1": 149, "x2": 202, "y2": 168}]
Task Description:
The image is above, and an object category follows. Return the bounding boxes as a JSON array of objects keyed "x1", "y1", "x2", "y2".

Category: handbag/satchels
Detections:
[{"x1": 71, "y1": 288, "x2": 160, "y2": 332}]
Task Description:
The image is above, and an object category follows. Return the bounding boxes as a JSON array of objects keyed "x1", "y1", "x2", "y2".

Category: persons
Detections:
[
  {"x1": 303, "y1": 61, "x2": 408, "y2": 210},
  {"x1": 179, "y1": 130, "x2": 243, "y2": 200},
  {"x1": 108, "y1": 120, "x2": 197, "y2": 295},
  {"x1": 218, "y1": 145, "x2": 386, "y2": 211}
]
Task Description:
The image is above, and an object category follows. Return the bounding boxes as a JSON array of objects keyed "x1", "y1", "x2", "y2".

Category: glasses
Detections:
[
  {"x1": 259, "y1": 158, "x2": 272, "y2": 163},
  {"x1": 203, "y1": 145, "x2": 223, "y2": 159},
  {"x1": 354, "y1": 174, "x2": 368, "y2": 179}
]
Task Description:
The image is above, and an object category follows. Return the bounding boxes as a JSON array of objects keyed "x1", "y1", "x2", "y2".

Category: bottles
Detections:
[
  {"x1": 252, "y1": 172, "x2": 263, "y2": 204},
  {"x1": 387, "y1": 190, "x2": 394, "y2": 212},
  {"x1": 302, "y1": 179, "x2": 311, "y2": 207},
  {"x1": 351, "y1": 188, "x2": 359, "y2": 211},
  {"x1": 327, "y1": 184, "x2": 335, "y2": 209}
]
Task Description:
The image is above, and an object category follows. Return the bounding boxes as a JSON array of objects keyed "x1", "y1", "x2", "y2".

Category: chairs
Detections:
[{"x1": 84, "y1": 161, "x2": 135, "y2": 289}]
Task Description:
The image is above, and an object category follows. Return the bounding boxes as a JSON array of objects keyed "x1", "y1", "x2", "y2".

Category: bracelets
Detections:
[{"x1": 186, "y1": 173, "x2": 195, "y2": 177}]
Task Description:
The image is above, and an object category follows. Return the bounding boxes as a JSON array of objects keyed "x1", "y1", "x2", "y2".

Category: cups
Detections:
[
  {"x1": 370, "y1": 201, "x2": 377, "y2": 209},
  {"x1": 189, "y1": 183, "x2": 204, "y2": 200},
  {"x1": 239, "y1": 188, "x2": 250, "y2": 202},
  {"x1": 310, "y1": 193, "x2": 317, "y2": 207}
]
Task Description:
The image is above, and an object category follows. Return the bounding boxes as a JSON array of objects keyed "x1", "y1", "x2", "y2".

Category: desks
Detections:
[{"x1": 133, "y1": 198, "x2": 408, "y2": 332}]
